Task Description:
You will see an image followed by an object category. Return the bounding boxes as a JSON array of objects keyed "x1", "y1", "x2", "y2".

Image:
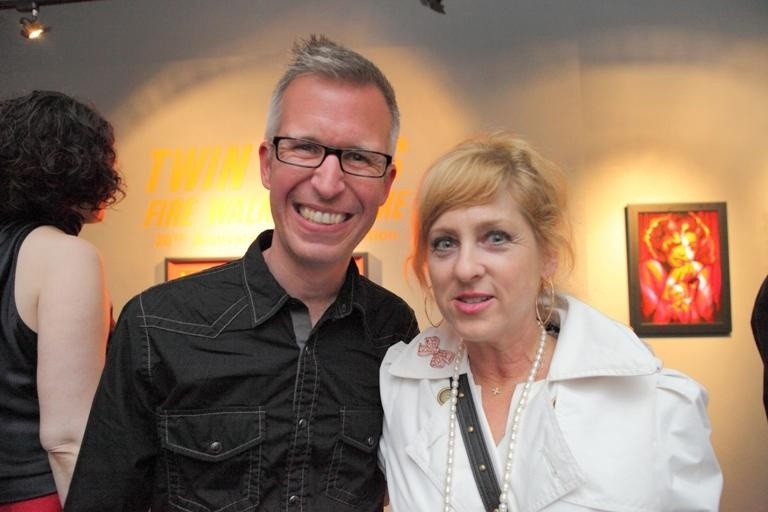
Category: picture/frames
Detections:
[
  {"x1": 623, "y1": 199, "x2": 734, "y2": 339},
  {"x1": 162, "y1": 251, "x2": 371, "y2": 408}
]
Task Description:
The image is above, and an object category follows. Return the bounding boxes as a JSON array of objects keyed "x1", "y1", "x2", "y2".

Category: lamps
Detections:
[{"x1": 16, "y1": 3, "x2": 53, "y2": 39}]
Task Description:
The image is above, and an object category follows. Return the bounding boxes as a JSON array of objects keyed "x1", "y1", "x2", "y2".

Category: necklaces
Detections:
[
  {"x1": 470, "y1": 360, "x2": 528, "y2": 395},
  {"x1": 440, "y1": 321, "x2": 551, "y2": 512}
]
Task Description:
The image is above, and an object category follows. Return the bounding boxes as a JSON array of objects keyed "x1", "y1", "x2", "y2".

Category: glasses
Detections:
[{"x1": 272, "y1": 138, "x2": 392, "y2": 178}]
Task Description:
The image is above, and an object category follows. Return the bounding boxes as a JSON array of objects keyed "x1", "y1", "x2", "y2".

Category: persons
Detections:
[
  {"x1": 59, "y1": 28, "x2": 424, "y2": 511},
  {"x1": 637, "y1": 212, "x2": 722, "y2": 325},
  {"x1": 373, "y1": 128, "x2": 730, "y2": 512},
  {"x1": 0, "y1": 85, "x2": 128, "y2": 512}
]
